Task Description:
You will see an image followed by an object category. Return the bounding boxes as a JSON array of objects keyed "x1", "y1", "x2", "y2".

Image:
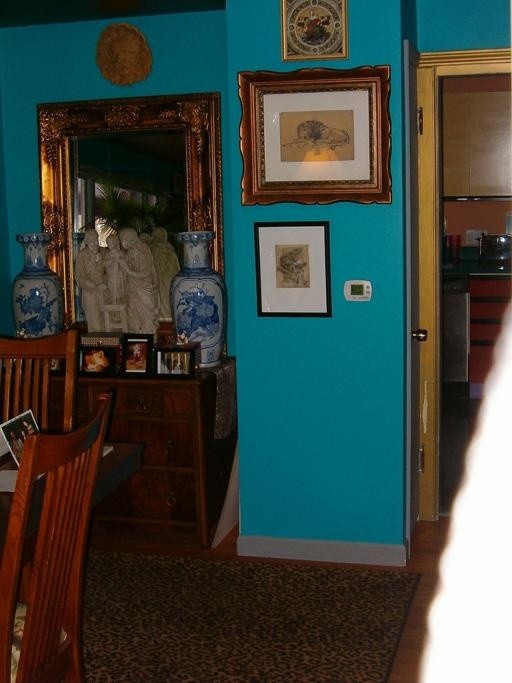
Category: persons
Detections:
[
  {"x1": 149, "y1": 226, "x2": 181, "y2": 319},
  {"x1": 74, "y1": 229, "x2": 106, "y2": 330},
  {"x1": 119, "y1": 226, "x2": 160, "y2": 332},
  {"x1": 138, "y1": 232, "x2": 151, "y2": 246},
  {"x1": 102, "y1": 233, "x2": 127, "y2": 304},
  {"x1": 7, "y1": 420, "x2": 38, "y2": 462}
]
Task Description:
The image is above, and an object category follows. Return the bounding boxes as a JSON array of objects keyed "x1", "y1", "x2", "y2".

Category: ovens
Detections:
[{"x1": 441, "y1": 278, "x2": 470, "y2": 383}]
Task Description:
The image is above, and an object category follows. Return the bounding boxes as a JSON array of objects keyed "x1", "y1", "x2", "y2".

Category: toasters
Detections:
[{"x1": 480, "y1": 233, "x2": 511, "y2": 261}]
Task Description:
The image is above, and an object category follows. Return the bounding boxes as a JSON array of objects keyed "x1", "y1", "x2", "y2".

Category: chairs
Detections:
[
  {"x1": 0, "y1": 388, "x2": 117, "y2": 683},
  {"x1": 1, "y1": 325, "x2": 84, "y2": 448}
]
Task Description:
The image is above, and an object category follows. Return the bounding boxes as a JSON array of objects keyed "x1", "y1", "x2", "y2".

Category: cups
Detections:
[{"x1": 449, "y1": 234, "x2": 461, "y2": 247}]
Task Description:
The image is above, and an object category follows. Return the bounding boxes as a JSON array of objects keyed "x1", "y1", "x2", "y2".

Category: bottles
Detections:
[{"x1": 156, "y1": 318, "x2": 176, "y2": 345}]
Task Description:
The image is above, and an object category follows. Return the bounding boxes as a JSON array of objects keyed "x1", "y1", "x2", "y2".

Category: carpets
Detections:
[{"x1": 82, "y1": 553, "x2": 421, "y2": 683}]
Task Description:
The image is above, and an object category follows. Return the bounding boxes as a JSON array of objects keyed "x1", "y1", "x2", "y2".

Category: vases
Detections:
[
  {"x1": 10, "y1": 231, "x2": 67, "y2": 371},
  {"x1": 170, "y1": 230, "x2": 228, "y2": 369}
]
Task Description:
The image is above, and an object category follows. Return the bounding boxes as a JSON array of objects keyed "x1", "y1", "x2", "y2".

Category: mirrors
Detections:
[{"x1": 36, "y1": 92, "x2": 224, "y2": 348}]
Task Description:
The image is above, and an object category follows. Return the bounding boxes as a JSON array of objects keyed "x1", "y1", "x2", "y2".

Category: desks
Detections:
[{"x1": 1, "y1": 437, "x2": 145, "y2": 573}]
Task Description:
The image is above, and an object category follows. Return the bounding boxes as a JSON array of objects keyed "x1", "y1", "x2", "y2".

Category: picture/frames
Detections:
[
  {"x1": 236, "y1": 63, "x2": 394, "y2": 204},
  {"x1": 79, "y1": 345, "x2": 115, "y2": 377},
  {"x1": 253, "y1": 220, "x2": 332, "y2": 319},
  {"x1": 157, "y1": 341, "x2": 202, "y2": 379},
  {"x1": 281, "y1": 0, "x2": 347, "y2": 60},
  {"x1": 121, "y1": 333, "x2": 154, "y2": 377}
]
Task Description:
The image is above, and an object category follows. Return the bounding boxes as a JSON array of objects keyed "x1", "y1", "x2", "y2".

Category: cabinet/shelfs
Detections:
[{"x1": 42, "y1": 354, "x2": 236, "y2": 549}]
